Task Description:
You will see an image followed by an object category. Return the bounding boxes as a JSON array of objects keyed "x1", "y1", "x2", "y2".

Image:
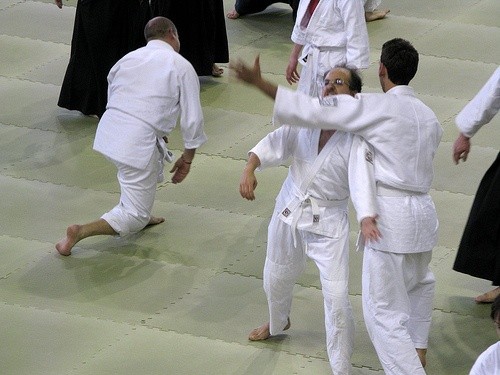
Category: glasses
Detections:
[{"x1": 324, "y1": 78, "x2": 351, "y2": 88}]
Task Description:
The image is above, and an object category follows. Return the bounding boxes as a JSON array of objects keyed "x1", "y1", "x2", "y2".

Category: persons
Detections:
[
  {"x1": 469, "y1": 293, "x2": 500, "y2": 375},
  {"x1": 239, "y1": 61, "x2": 384, "y2": 375},
  {"x1": 55, "y1": 16, "x2": 209, "y2": 257},
  {"x1": 225, "y1": 0, "x2": 299, "y2": 24},
  {"x1": 285, "y1": 0, "x2": 372, "y2": 95},
  {"x1": 364, "y1": 0, "x2": 391, "y2": 22},
  {"x1": 57, "y1": 0, "x2": 230, "y2": 119},
  {"x1": 452, "y1": 63, "x2": 500, "y2": 303},
  {"x1": 228, "y1": 38, "x2": 443, "y2": 375}
]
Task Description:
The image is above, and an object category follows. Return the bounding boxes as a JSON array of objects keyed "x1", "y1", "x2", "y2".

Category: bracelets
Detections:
[{"x1": 181, "y1": 154, "x2": 192, "y2": 165}]
